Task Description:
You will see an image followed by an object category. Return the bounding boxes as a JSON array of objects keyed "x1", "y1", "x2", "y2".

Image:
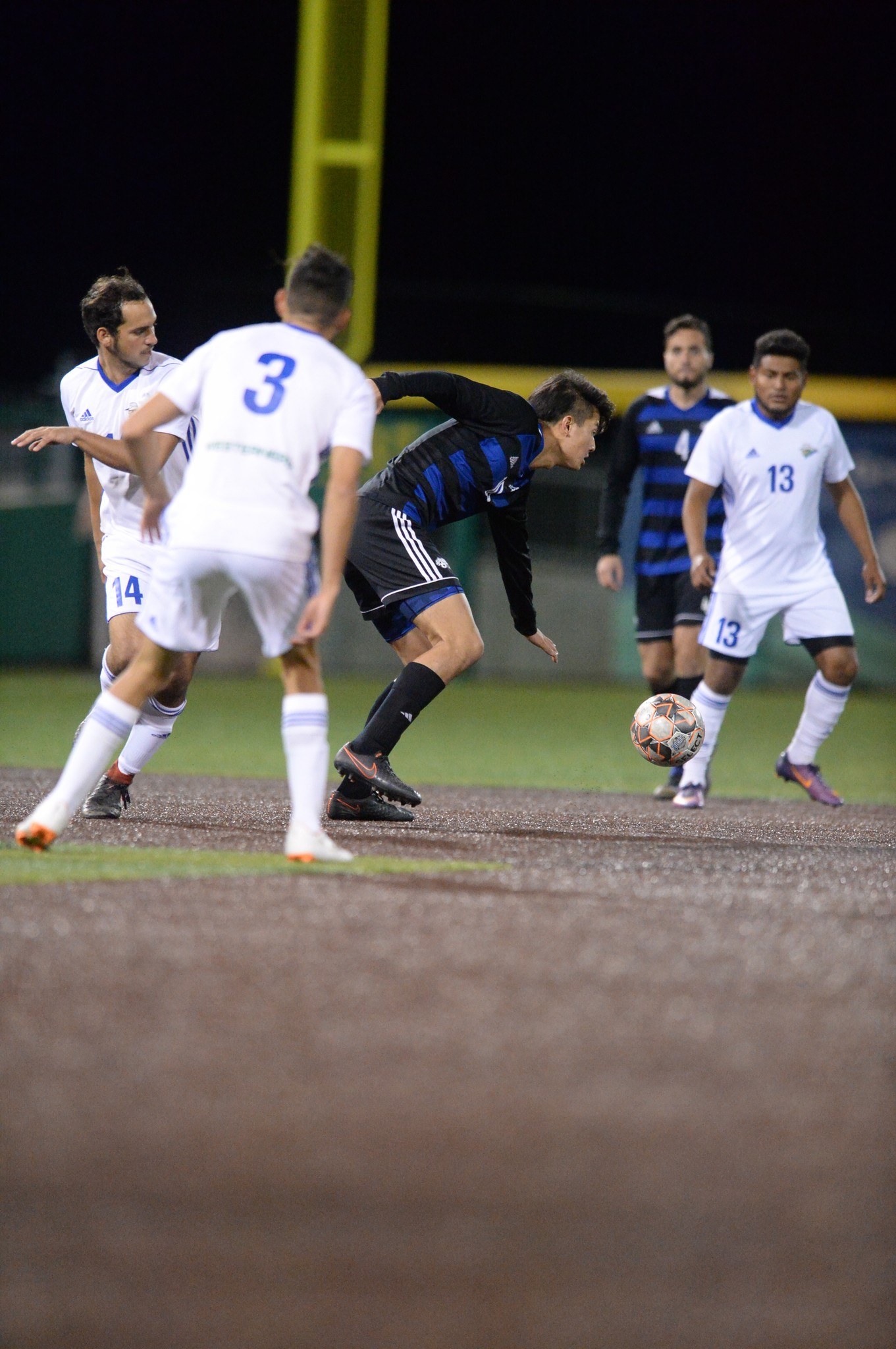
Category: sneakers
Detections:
[
  {"x1": 672, "y1": 775, "x2": 711, "y2": 808},
  {"x1": 655, "y1": 777, "x2": 680, "y2": 799},
  {"x1": 326, "y1": 790, "x2": 415, "y2": 823},
  {"x1": 81, "y1": 770, "x2": 132, "y2": 820},
  {"x1": 15, "y1": 801, "x2": 70, "y2": 853},
  {"x1": 282, "y1": 825, "x2": 355, "y2": 864},
  {"x1": 333, "y1": 741, "x2": 422, "y2": 808},
  {"x1": 775, "y1": 750, "x2": 845, "y2": 809}
]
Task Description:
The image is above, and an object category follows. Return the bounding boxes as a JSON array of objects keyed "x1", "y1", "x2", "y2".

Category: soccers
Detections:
[{"x1": 631, "y1": 693, "x2": 704, "y2": 767}]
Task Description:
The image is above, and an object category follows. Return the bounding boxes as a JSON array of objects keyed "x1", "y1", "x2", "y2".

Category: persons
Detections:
[
  {"x1": 325, "y1": 364, "x2": 616, "y2": 827},
  {"x1": 12, "y1": 237, "x2": 378, "y2": 867},
  {"x1": 669, "y1": 328, "x2": 889, "y2": 809},
  {"x1": 10, "y1": 277, "x2": 201, "y2": 823},
  {"x1": 594, "y1": 314, "x2": 744, "y2": 805}
]
